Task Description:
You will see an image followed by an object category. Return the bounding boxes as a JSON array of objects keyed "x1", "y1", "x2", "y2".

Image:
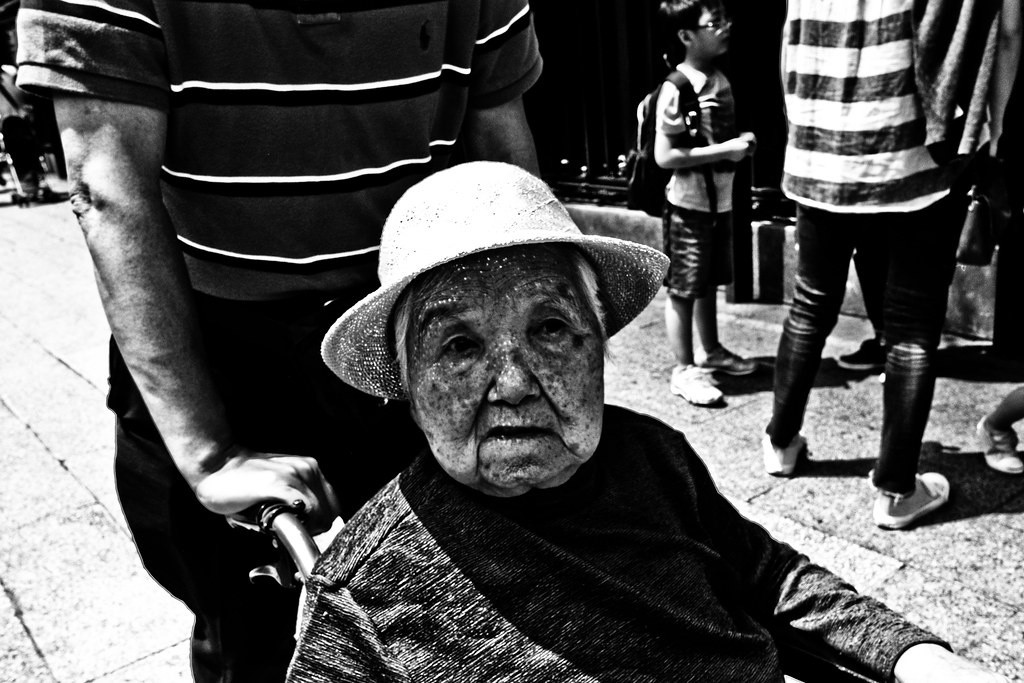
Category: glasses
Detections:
[{"x1": 692, "y1": 16, "x2": 721, "y2": 30}]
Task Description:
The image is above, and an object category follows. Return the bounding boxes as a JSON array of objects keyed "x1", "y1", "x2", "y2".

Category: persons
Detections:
[
  {"x1": 286, "y1": 160, "x2": 1014, "y2": 683},
  {"x1": 16, "y1": 0, "x2": 543, "y2": 683},
  {"x1": 0, "y1": 0, "x2": 1024, "y2": 530}
]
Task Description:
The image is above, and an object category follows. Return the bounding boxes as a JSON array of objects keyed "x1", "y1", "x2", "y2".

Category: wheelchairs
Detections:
[{"x1": 0, "y1": 130, "x2": 66, "y2": 206}]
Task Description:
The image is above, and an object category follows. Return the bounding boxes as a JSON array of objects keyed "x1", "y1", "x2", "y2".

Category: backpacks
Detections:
[{"x1": 636, "y1": 69, "x2": 720, "y2": 234}]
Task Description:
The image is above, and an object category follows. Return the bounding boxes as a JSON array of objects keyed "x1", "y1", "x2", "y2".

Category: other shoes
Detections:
[
  {"x1": 868, "y1": 468, "x2": 950, "y2": 529},
  {"x1": 761, "y1": 430, "x2": 802, "y2": 474}
]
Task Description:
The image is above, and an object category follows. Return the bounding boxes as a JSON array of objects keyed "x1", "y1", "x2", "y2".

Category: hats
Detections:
[{"x1": 320, "y1": 160, "x2": 673, "y2": 401}]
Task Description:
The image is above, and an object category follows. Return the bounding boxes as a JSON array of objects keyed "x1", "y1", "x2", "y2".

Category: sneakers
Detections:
[
  {"x1": 695, "y1": 343, "x2": 756, "y2": 375},
  {"x1": 670, "y1": 366, "x2": 724, "y2": 405},
  {"x1": 976, "y1": 414, "x2": 1024, "y2": 474}
]
